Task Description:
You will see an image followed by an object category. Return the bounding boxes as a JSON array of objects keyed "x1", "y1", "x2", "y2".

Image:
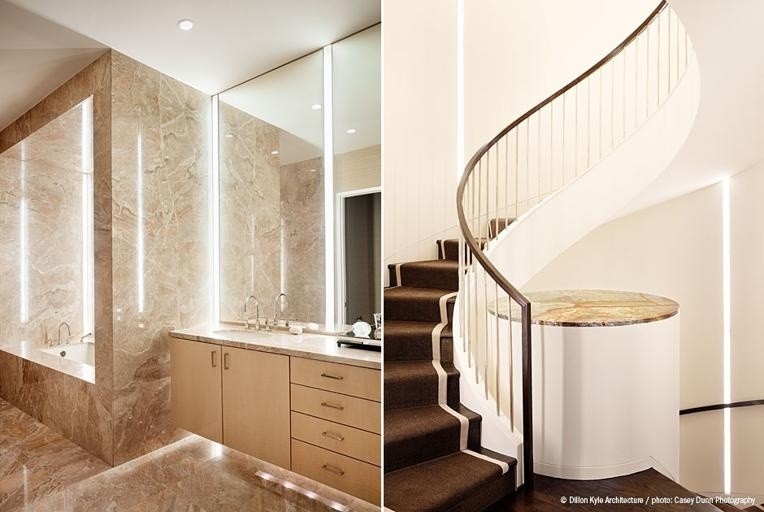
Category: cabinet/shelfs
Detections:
[{"x1": 166, "y1": 338, "x2": 381, "y2": 512}]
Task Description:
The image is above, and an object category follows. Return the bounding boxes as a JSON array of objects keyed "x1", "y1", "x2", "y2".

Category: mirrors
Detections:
[
  {"x1": 337, "y1": 184, "x2": 381, "y2": 336},
  {"x1": 215, "y1": 44, "x2": 326, "y2": 333},
  {"x1": 331, "y1": 22, "x2": 382, "y2": 193}
]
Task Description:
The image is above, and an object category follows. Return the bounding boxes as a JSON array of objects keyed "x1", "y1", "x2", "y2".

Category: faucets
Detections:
[
  {"x1": 45, "y1": 334, "x2": 53, "y2": 347},
  {"x1": 57, "y1": 322, "x2": 71, "y2": 345},
  {"x1": 273, "y1": 292, "x2": 290, "y2": 326},
  {"x1": 243, "y1": 295, "x2": 260, "y2": 331}
]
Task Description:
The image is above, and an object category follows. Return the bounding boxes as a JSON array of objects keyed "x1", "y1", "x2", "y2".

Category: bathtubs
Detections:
[{"x1": 39, "y1": 340, "x2": 95, "y2": 370}]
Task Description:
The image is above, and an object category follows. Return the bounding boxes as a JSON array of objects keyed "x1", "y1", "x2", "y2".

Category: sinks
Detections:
[
  {"x1": 212, "y1": 328, "x2": 271, "y2": 340},
  {"x1": 278, "y1": 320, "x2": 303, "y2": 327}
]
